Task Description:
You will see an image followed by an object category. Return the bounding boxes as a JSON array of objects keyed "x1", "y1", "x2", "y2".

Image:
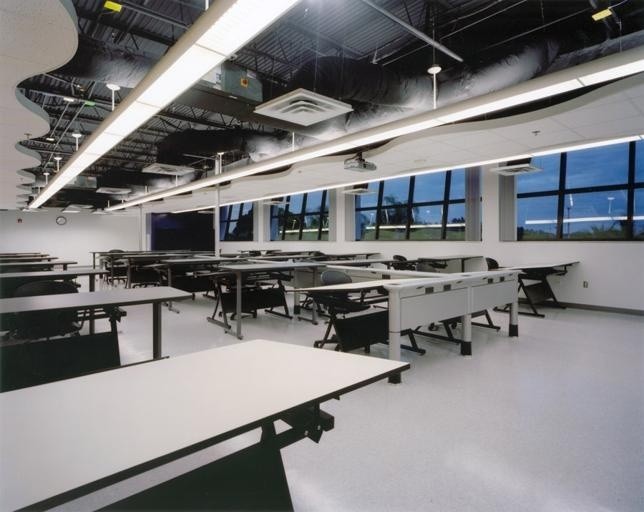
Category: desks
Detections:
[
  {"x1": 390, "y1": 254, "x2": 579, "y2": 357},
  {"x1": 1, "y1": 340, "x2": 411, "y2": 510},
  {"x1": 0, "y1": 250, "x2": 437, "y2": 388}
]
